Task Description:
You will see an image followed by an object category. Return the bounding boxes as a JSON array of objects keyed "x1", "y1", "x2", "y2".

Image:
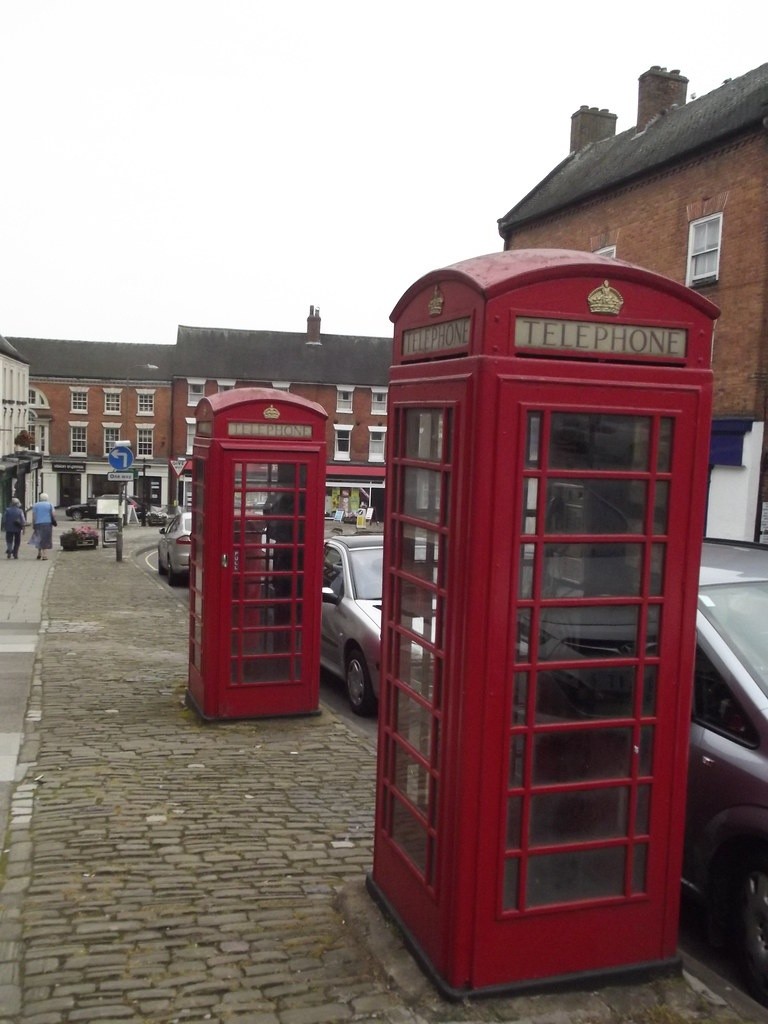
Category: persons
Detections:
[
  {"x1": 533, "y1": 424, "x2": 654, "y2": 815},
  {"x1": 1, "y1": 497, "x2": 25, "y2": 560},
  {"x1": 262, "y1": 483, "x2": 304, "y2": 671},
  {"x1": 27, "y1": 493, "x2": 56, "y2": 560}
]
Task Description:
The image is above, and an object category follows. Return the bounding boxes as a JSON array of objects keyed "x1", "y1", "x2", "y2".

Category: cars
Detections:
[
  {"x1": 66, "y1": 494, "x2": 145, "y2": 521},
  {"x1": 321, "y1": 534, "x2": 448, "y2": 718},
  {"x1": 515, "y1": 485, "x2": 666, "y2": 737},
  {"x1": 157, "y1": 512, "x2": 192, "y2": 586},
  {"x1": 679, "y1": 538, "x2": 768, "y2": 1010}
]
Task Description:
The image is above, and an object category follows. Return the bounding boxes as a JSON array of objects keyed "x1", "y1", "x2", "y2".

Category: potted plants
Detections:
[
  {"x1": 60, "y1": 527, "x2": 99, "y2": 551},
  {"x1": 146, "y1": 511, "x2": 168, "y2": 526}
]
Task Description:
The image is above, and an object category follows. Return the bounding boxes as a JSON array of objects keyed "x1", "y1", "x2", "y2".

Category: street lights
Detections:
[{"x1": 141, "y1": 458, "x2": 150, "y2": 526}]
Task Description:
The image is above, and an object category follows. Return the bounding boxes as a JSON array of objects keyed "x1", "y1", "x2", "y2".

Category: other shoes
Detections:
[
  {"x1": 37, "y1": 553, "x2": 41, "y2": 559},
  {"x1": 12, "y1": 550, "x2": 18, "y2": 559},
  {"x1": 42, "y1": 556, "x2": 47, "y2": 560},
  {"x1": 6, "y1": 549, "x2": 11, "y2": 559}
]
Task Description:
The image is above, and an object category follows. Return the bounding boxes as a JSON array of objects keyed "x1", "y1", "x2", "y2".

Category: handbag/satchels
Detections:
[
  {"x1": 49, "y1": 504, "x2": 58, "y2": 527},
  {"x1": 14, "y1": 508, "x2": 22, "y2": 529}
]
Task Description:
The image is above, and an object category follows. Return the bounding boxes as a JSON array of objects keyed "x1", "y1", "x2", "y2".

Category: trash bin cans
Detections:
[{"x1": 101, "y1": 516, "x2": 122, "y2": 548}]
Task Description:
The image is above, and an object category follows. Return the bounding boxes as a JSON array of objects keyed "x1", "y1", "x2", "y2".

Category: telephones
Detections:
[
  {"x1": 261, "y1": 483, "x2": 293, "y2": 543},
  {"x1": 533, "y1": 481, "x2": 630, "y2": 589}
]
